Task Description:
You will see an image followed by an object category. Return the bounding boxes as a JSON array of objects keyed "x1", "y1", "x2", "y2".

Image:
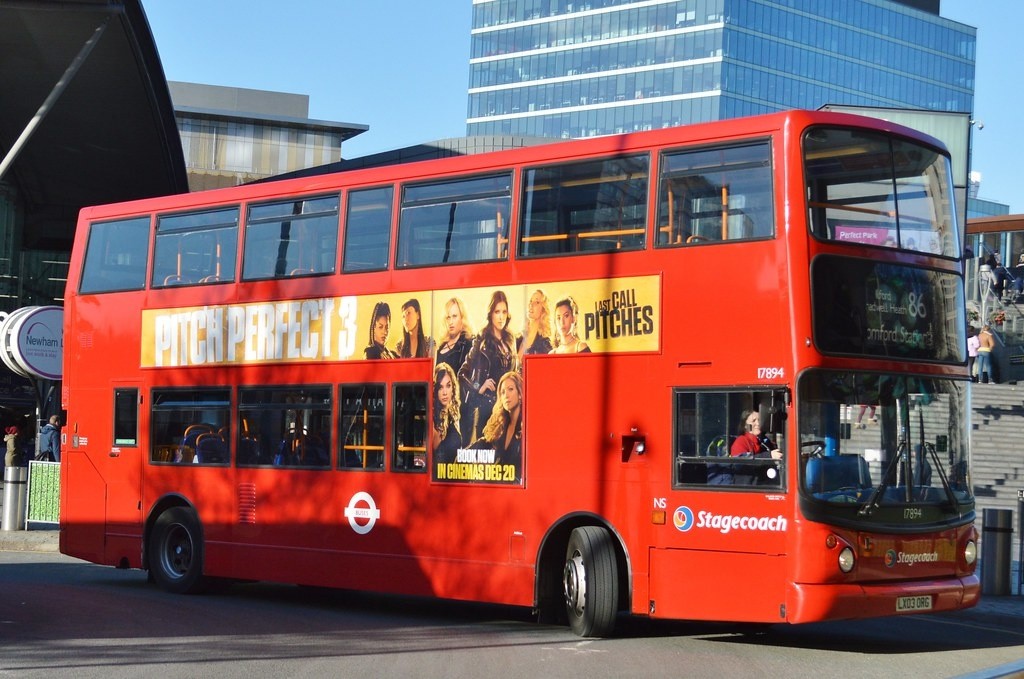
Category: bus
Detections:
[{"x1": 58, "y1": 109, "x2": 982, "y2": 639}]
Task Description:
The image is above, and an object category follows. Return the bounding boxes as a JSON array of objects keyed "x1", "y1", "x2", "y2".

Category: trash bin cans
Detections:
[{"x1": 979, "y1": 507, "x2": 1013, "y2": 597}]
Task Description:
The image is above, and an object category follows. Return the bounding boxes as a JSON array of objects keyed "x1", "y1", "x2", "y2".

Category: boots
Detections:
[
  {"x1": 855, "y1": 422, "x2": 861, "y2": 428},
  {"x1": 868, "y1": 419, "x2": 877, "y2": 425}
]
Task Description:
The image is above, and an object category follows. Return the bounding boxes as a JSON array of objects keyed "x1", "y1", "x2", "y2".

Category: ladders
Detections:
[{"x1": 1, "y1": 466, "x2": 28, "y2": 531}]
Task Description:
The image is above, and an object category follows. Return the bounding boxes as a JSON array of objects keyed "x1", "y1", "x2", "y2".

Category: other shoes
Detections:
[
  {"x1": 988, "y1": 381, "x2": 996, "y2": 384},
  {"x1": 978, "y1": 382, "x2": 984, "y2": 385}
]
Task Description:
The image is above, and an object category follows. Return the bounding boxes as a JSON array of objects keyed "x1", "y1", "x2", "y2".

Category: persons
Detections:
[
  {"x1": 434, "y1": 361, "x2": 463, "y2": 482},
  {"x1": 730, "y1": 407, "x2": 784, "y2": 486},
  {"x1": 914, "y1": 393, "x2": 930, "y2": 410},
  {"x1": 978, "y1": 262, "x2": 997, "y2": 300},
  {"x1": 1011, "y1": 276, "x2": 1024, "y2": 304},
  {"x1": 396, "y1": 298, "x2": 435, "y2": 387},
  {"x1": 977, "y1": 325, "x2": 997, "y2": 384},
  {"x1": 362, "y1": 304, "x2": 400, "y2": 358},
  {"x1": 40, "y1": 415, "x2": 60, "y2": 462},
  {"x1": 990, "y1": 262, "x2": 1007, "y2": 302},
  {"x1": 966, "y1": 326, "x2": 980, "y2": 382},
  {"x1": 516, "y1": 289, "x2": 552, "y2": 371},
  {"x1": 986, "y1": 253, "x2": 997, "y2": 270},
  {"x1": 913, "y1": 444, "x2": 932, "y2": 500},
  {"x1": 547, "y1": 298, "x2": 592, "y2": 354},
  {"x1": 856, "y1": 405, "x2": 878, "y2": 425},
  {"x1": 437, "y1": 297, "x2": 478, "y2": 448},
  {"x1": 3, "y1": 426, "x2": 22, "y2": 467},
  {"x1": 458, "y1": 291, "x2": 516, "y2": 440},
  {"x1": 454, "y1": 371, "x2": 523, "y2": 485}
]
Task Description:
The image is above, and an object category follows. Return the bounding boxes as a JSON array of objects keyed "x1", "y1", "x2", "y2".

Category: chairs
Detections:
[
  {"x1": 173, "y1": 424, "x2": 361, "y2": 467},
  {"x1": 706, "y1": 435, "x2": 741, "y2": 486},
  {"x1": 164, "y1": 268, "x2": 314, "y2": 286}
]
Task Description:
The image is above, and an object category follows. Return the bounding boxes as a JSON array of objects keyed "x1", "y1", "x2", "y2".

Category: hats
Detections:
[{"x1": 5, "y1": 426, "x2": 18, "y2": 435}]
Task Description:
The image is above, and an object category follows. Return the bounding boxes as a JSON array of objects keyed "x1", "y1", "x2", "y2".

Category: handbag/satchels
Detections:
[{"x1": 34, "y1": 451, "x2": 53, "y2": 461}]
